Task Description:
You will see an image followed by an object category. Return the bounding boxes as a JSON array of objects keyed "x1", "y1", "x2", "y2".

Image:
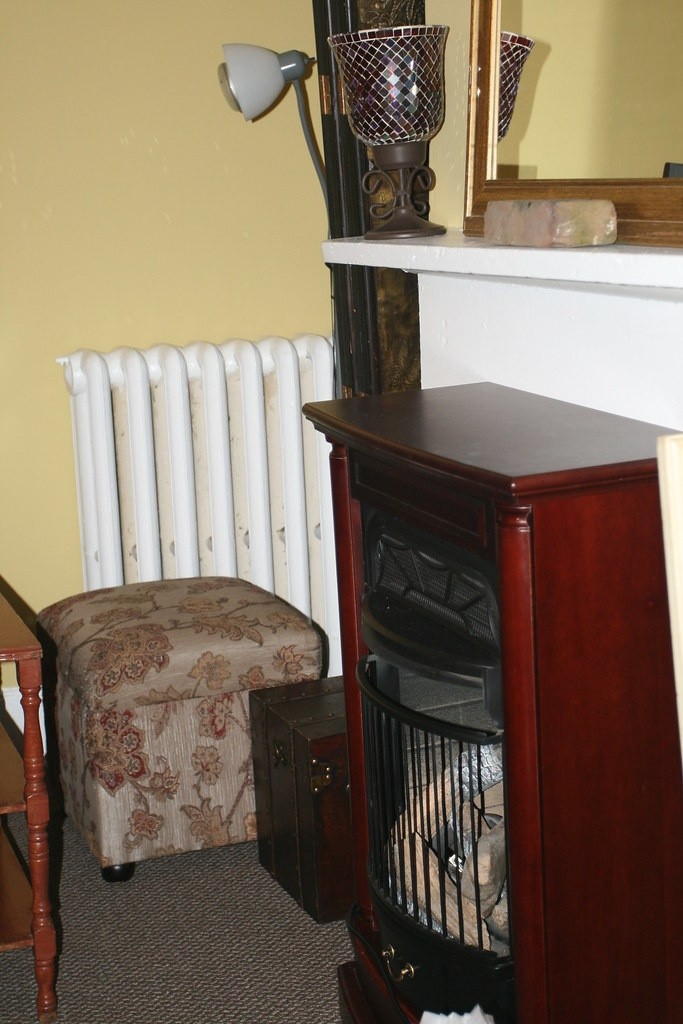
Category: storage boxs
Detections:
[{"x1": 249, "y1": 676, "x2": 352, "y2": 924}]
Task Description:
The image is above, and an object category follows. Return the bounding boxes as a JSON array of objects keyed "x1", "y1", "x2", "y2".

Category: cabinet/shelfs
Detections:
[
  {"x1": 301, "y1": 381, "x2": 682, "y2": 1024},
  {"x1": 0, "y1": 590, "x2": 58, "y2": 1024}
]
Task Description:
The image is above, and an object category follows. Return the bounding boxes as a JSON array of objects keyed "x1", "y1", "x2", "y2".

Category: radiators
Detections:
[{"x1": 56, "y1": 333, "x2": 343, "y2": 677}]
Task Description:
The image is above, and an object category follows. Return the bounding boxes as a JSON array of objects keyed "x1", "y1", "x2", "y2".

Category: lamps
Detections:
[
  {"x1": 220, "y1": 42, "x2": 346, "y2": 400},
  {"x1": 327, "y1": 24, "x2": 450, "y2": 239}
]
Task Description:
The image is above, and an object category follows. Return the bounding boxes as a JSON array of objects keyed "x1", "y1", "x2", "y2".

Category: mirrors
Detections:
[{"x1": 461, "y1": 0, "x2": 683, "y2": 249}]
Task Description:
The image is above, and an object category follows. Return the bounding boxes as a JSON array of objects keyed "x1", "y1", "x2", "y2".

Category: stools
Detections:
[{"x1": 36, "y1": 577, "x2": 324, "y2": 883}]
